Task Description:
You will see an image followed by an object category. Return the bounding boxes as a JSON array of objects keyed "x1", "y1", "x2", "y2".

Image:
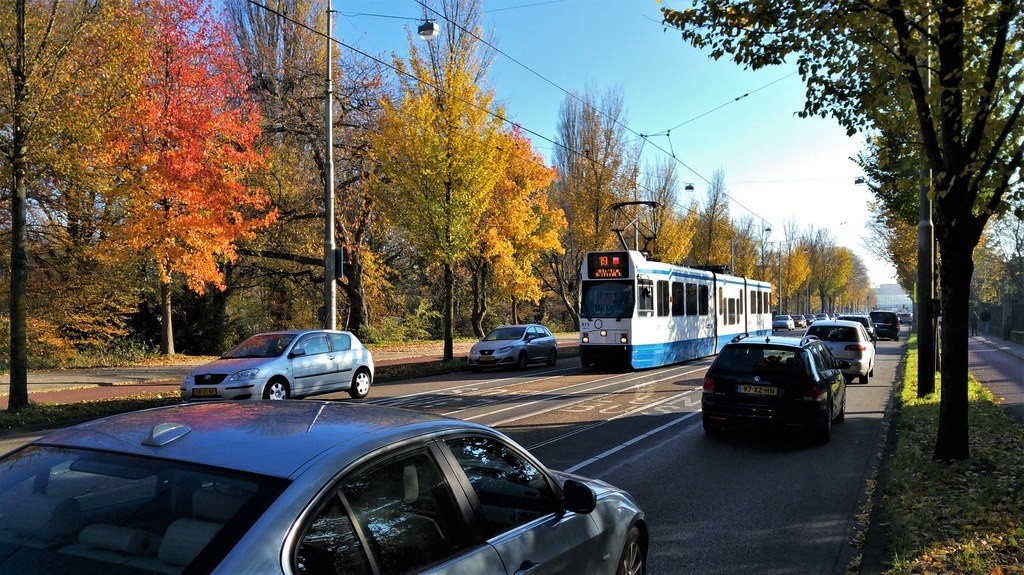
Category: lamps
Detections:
[
  {"x1": 803, "y1": 248, "x2": 809, "y2": 253},
  {"x1": 765, "y1": 227, "x2": 771, "y2": 232},
  {"x1": 685, "y1": 183, "x2": 694, "y2": 192},
  {"x1": 417, "y1": 18, "x2": 439, "y2": 40}
]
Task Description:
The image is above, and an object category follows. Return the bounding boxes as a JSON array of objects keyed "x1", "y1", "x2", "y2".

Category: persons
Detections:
[
  {"x1": 969, "y1": 311, "x2": 980, "y2": 336},
  {"x1": 979, "y1": 306, "x2": 991, "y2": 336}
]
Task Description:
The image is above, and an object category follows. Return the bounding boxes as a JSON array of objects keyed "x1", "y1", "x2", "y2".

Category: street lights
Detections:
[{"x1": 762, "y1": 227, "x2": 771, "y2": 281}]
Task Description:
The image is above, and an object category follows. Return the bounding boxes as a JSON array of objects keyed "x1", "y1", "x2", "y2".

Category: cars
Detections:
[
  {"x1": 804, "y1": 312, "x2": 871, "y2": 326},
  {"x1": 772, "y1": 314, "x2": 795, "y2": 331},
  {"x1": 180, "y1": 328, "x2": 374, "y2": 401},
  {"x1": 0, "y1": 398, "x2": 653, "y2": 575},
  {"x1": 468, "y1": 324, "x2": 559, "y2": 372},
  {"x1": 888, "y1": 306, "x2": 913, "y2": 325},
  {"x1": 791, "y1": 315, "x2": 807, "y2": 329}
]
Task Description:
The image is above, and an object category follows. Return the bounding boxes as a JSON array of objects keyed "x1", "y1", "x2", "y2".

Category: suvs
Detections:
[
  {"x1": 869, "y1": 310, "x2": 904, "y2": 341},
  {"x1": 701, "y1": 332, "x2": 852, "y2": 446},
  {"x1": 799, "y1": 319, "x2": 879, "y2": 384},
  {"x1": 836, "y1": 314, "x2": 879, "y2": 350}
]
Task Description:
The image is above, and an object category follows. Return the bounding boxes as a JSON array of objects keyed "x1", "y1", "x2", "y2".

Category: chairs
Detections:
[
  {"x1": 819, "y1": 328, "x2": 830, "y2": 337},
  {"x1": 80, "y1": 524, "x2": 145, "y2": 551},
  {"x1": 360, "y1": 459, "x2": 419, "y2": 503},
  {"x1": 749, "y1": 349, "x2": 765, "y2": 357},
  {"x1": 191, "y1": 490, "x2": 232, "y2": 517},
  {"x1": 13, "y1": 492, "x2": 82, "y2": 538},
  {"x1": 159, "y1": 517, "x2": 225, "y2": 562}
]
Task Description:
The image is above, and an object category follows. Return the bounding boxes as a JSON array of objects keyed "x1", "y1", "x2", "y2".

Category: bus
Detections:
[{"x1": 567, "y1": 200, "x2": 774, "y2": 372}]
